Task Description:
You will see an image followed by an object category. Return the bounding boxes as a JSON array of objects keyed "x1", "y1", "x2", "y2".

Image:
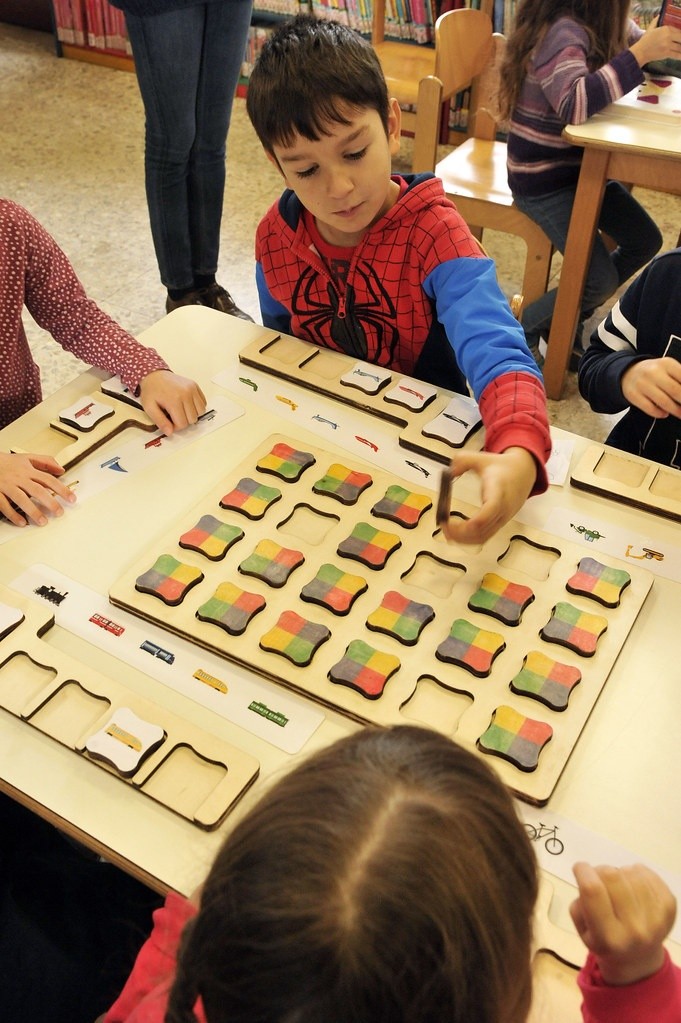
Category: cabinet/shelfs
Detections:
[{"x1": 53, "y1": 0, "x2": 506, "y2": 146}]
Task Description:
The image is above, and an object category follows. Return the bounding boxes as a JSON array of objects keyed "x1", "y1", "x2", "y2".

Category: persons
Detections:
[
  {"x1": 106, "y1": 0, "x2": 256, "y2": 325},
  {"x1": 472, "y1": 0, "x2": 681, "y2": 373},
  {"x1": 0, "y1": 198, "x2": 208, "y2": 529},
  {"x1": 94, "y1": 724, "x2": 681, "y2": 1023},
  {"x1": 576, "y1": 250, "x2": 681, "y2": 472},
  {"x1": 246, "y1": 14, "x2": 554, "y2": 545}
]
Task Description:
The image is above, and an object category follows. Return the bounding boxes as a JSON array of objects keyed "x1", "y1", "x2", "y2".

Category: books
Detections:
[
  {"x1": 649, "y1": 0, "x2": 681, "y2": 78},
  {"x1": 53, "y1": 0, "x2": 527, "y2": 79}
]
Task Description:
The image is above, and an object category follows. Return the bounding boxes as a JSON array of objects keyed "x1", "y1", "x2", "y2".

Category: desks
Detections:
[
  {"x1": 0, "y1": 303, "x2": 681, "y2": 1023},
  {"x1": 544, "y1": 73, "x2": 681, "y2": 403}
]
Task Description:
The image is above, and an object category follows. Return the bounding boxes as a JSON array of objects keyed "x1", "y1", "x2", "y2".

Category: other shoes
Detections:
[
  {"x1": 165, "y1": 290, "x2": 206, "y2": 315},
  {"x1": 200, "y1": 283, "x2": 255, "y2": 323},
  {"x1": 570, "y1": 322, "x2": 586, "y2": 370},
  {"x1": 525, "y1": 331, "x2": 545, "y2": 367}
]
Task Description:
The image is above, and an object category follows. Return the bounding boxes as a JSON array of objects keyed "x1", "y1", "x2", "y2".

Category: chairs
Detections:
[
  {"x1": 410, "y1": 8, "x2": 556, "y2": 323},
  {"x1": 369, "y1": 0, "x2": 495, "y2": 106}
]
